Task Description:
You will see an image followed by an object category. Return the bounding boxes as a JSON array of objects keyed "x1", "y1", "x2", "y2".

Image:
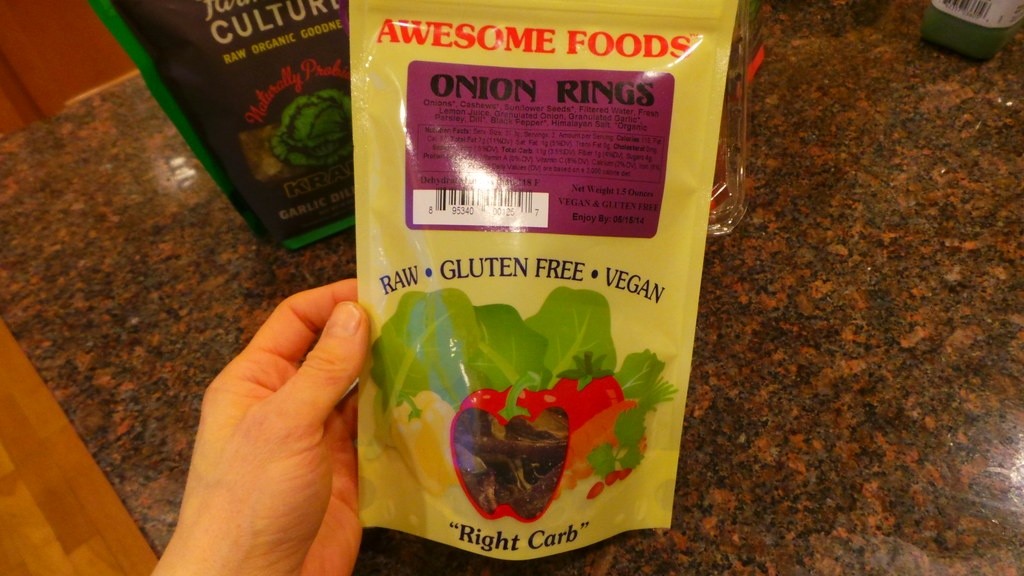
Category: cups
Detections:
[{"x1": 702, "y1": 0, "x2": 755, "y2": 236}]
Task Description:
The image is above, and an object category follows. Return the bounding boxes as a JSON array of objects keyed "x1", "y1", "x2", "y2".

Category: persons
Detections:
[{"x1": 151, "y1": 279, "x2": 371, "y2": 576}]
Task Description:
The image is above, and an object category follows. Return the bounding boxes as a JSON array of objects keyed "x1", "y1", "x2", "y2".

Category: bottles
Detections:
[{"x1": 919, "y1": 0, "x2": 1024, "y2": 60}]
